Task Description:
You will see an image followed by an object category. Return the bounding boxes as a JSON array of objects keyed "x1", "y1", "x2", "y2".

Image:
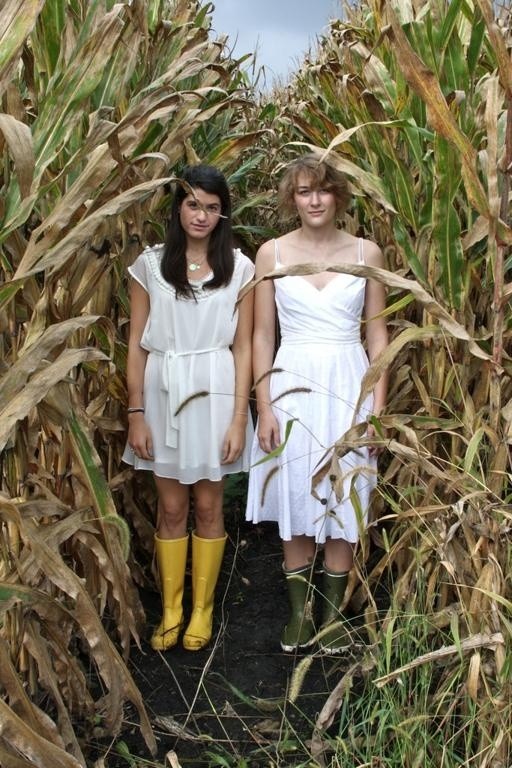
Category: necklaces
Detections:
[{"x1": 182, "y1": 250, "x2": 208, "y2": 272}]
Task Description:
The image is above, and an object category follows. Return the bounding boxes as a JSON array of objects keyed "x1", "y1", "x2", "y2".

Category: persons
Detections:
[
  {"x1": 254, "y1": 156, "x2": 390, "y2": 655},
  {"x1": 120, "y1": 167, "x2": 254, "y2": 654}
]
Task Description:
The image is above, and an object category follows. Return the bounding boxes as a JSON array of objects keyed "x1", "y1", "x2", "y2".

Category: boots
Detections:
[
  {"x1": 182, "y1": 528, "x2": 229, "y2": 651},
  {"x1": 150, "y1": 533, "x2": 189, "y2": 651},
  {"x1": 317, "y1": 563, "x2": 355, "y2": 655},
  {"x1": 279, "y1": 562, "x2": 316, "y2": 654}
]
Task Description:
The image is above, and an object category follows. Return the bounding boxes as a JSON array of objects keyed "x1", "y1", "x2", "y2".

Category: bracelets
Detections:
[{"x1": 127, "y1": 406, "x2": 145, "y2": 414}]
